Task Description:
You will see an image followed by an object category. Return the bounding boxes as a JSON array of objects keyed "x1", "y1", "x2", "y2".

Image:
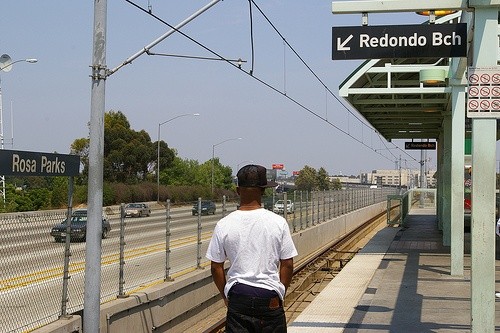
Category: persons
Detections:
[{"x1": 204, "y1": 164, "x2": 298, "y2": 333}]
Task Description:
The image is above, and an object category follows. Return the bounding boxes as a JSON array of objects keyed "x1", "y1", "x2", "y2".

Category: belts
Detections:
[{"x1": 230, "y1": 294, "x2": 282, "y2": 307}]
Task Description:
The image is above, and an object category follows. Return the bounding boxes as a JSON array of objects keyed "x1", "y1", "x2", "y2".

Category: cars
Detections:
[
  {"x1": 124, "y1": 203, "x2": 151, "y2": 217},
  {"x1": 50, "y1": 209, "x2": 111, "y2": 243},
  {"x1": 273, "y1": 200, "x2": 295, "y2": 214},
  {"x1": 192, "y1": 199, "x2": 217, "y2": 216},
  {"x1": 264, "y1": 196, "x2": 279, "y2": 209}
]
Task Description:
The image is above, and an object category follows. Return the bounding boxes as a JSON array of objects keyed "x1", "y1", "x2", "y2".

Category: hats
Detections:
[{"x1": 236, "y1": 164, "x2": 278, "y2": 188}]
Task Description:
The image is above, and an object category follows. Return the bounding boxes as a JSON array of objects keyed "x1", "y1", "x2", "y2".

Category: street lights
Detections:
[
  {"x1": 211, "y1": 137, "x2": 243, "y2": 199},
  {"x1": 157, "y1": 113, "x2": 200, "y2": 202}
]
